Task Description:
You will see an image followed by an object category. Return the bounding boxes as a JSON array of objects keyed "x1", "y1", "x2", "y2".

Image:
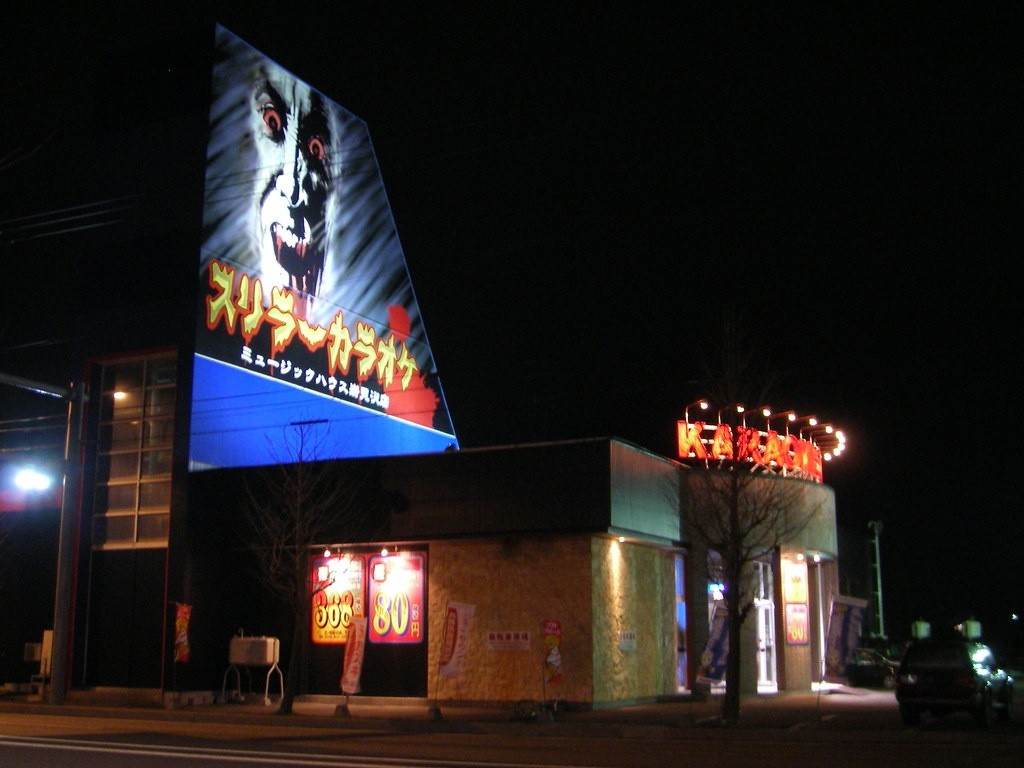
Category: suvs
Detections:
[{"x1": 895, "y1": 636, "x2": 1015, "y2": 728}]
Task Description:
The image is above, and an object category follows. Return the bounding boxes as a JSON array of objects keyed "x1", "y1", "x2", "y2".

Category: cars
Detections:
[{"x1": 848, "y1": 647, "x2": 900, "y2": 690}]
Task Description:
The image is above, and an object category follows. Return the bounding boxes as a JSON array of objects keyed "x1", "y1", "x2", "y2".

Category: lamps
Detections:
[
  {"x1": 381, "y1": 545, "x2": 388, "y2": 557},
  {"x1": 324, "y1": 546, "x2": 331, "y2": 558},
  {"x1": 685, "y1": 399, "x2": 847, "y2": 461}
]
